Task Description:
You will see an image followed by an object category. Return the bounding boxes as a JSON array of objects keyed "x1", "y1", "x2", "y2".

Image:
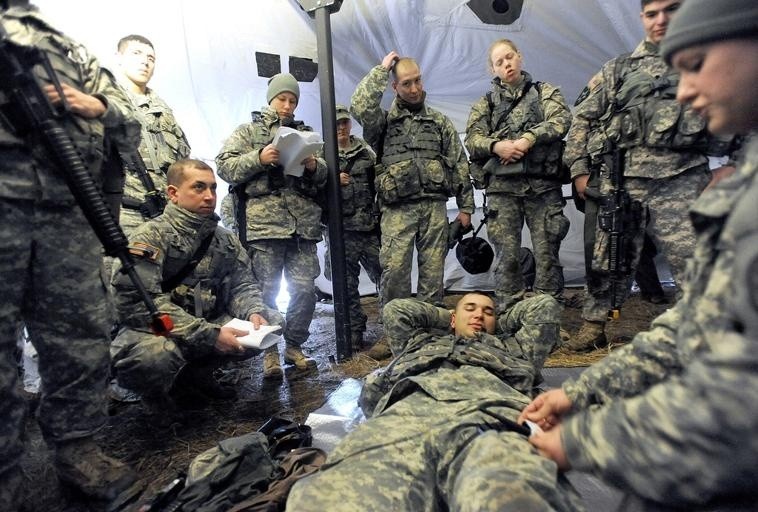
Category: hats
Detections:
[
  {"x1": 661, "y1": 0, "x2": 758, "y2": 70},
  {"x1": 448, "y1": 220, "x2": 475, "y2": 244},
  {"x1": 336, "y1": 104, "x2": 350, "y2": 120},
  {"x1": 267, "y1": 72, "x2": 299, "y2": 105}
]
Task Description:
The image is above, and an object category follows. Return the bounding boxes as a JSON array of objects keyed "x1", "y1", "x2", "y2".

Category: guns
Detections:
[
  {"x1": 2, "y1": 38, "x2": 175, "y2": 337},
  {"x1": 122, "y1": 148, "x2": 167, "y2": 219},
  {"x1": 588, "y1": 133, "x2": 629, "y2": 316}
]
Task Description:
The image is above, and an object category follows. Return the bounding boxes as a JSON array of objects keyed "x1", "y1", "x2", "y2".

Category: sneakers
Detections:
[
  {"x1": 263, "y1": 344, "x2": 284, "y2": 378},
  {"x1": 51, "y1": 434, "x2": 137, "y2": 499},
  {"x1": 187, "y1": 368, "x2": 236, "y2": 404},
  {"x1": 351, "y1": 330, "x2": 364, "y2": 351},
  {"x1": 139, "y1": 392, "x2": 185, "y2": 421},
  {"x1": 283, "y1": 346, "x2": 317, "y2": 371},
  {"x1": 369, "y1": 336, "x2": 392, "y2": 360},
  {"x1": 563, "y1": 322, "x2": 608, "y2": 352},
  {"x1": 641, "y1": 284, "x2": 669, "y2": 304}
]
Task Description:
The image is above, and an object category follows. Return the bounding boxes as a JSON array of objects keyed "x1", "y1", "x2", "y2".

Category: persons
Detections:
[
  {"x1": 283, "y1": 289, "x2": 565, "y2": 510},
  {"x1": 2, "y1": 0, "x2": 144, "y2": 510},
  {"x1": 513, "y1": 0, "x2": 757, "y2": 509},
  {"x1": 0, "y1": 0, "x2": 758, "y2": 429}
]
